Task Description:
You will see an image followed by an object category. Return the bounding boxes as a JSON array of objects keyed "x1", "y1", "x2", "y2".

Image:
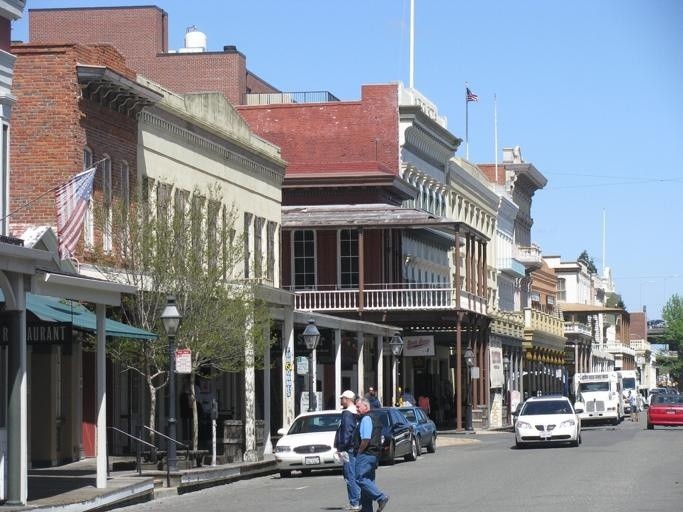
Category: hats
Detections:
[{"x1": 339, "y1": 390, "x2": 358, "y2": 400}]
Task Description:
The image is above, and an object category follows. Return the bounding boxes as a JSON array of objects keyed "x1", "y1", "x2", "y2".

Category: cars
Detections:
[
  {"x1": 514, "y1": 369, "x2": 683, "y2": 447},
  {"x1": 274, "y1": 405, "x2": 439, "y2": 476}
]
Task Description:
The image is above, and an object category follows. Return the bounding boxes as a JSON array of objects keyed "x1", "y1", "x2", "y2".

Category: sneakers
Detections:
[
  {"x1": 345, "y1": 504, "x2": 364, "y2": 512},
  {"x1": 376, "y1": 494, "x2": 392, "y2": 511}
]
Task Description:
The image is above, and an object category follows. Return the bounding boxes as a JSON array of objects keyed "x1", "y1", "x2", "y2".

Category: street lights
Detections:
[
  {"x1": 299, "y1": 317, "x2": 319, "y2": 411},
  {"x1": 159, "y1": 293, "x2": 185, "y2": 470},
  {"x1": 461, "y1": 347, "x2": 479, "y2": 437},
  {"x1": 387, "y1": 331, "x2": 404, "y2": 406}
]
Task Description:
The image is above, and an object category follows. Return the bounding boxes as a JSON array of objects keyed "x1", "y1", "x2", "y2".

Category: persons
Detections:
[
  {"x1": 353, "y1": 396, "x2": 390, "y2": 512},
  {"x1": 418, "y1": 390, "x2": 431, "y2": 415},
  {"x1": 365, "y1": 386, "x2": 381, "y2": 409},
  {"x1": 400, "y1": 387, "x2": 417, "y2": 405},
  {"x1": 55, "y1": 166, "x2": 97, "y2": 261},
  {"x1": 334, "y1": 390, "x2": 362, "y2": 510},
  {"x1": 623, "y1": 390, "x2": 639, "y2": 422}
]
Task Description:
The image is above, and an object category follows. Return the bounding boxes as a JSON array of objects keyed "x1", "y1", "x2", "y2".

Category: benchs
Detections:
[{"x1": 138, "y1": 448, "x2": 208, "y2": 469}]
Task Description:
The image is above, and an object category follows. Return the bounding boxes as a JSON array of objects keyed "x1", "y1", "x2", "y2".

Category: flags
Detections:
[{"x1": 467, "y1": 88, "x2": 479, "y2": 105}]
